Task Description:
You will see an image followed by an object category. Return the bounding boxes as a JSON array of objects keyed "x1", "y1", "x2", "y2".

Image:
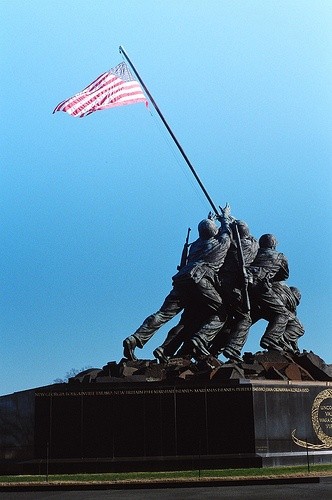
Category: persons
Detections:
[{"x1": 121, "y1": 203, "x2": 304, "y2": 361}]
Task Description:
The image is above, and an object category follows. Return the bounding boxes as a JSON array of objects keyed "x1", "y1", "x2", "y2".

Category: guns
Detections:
[
  {"x1": 176, "y1": 227, "x2": 191, "y2": 271},
  {"x1": 233, "y1": 221, "x2": 251, "y2": 317}
]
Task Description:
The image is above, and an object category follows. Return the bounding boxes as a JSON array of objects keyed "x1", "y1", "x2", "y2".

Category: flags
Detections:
[{"x1": 53, "y1": 60, "x2": 148, "y2": 117}]
